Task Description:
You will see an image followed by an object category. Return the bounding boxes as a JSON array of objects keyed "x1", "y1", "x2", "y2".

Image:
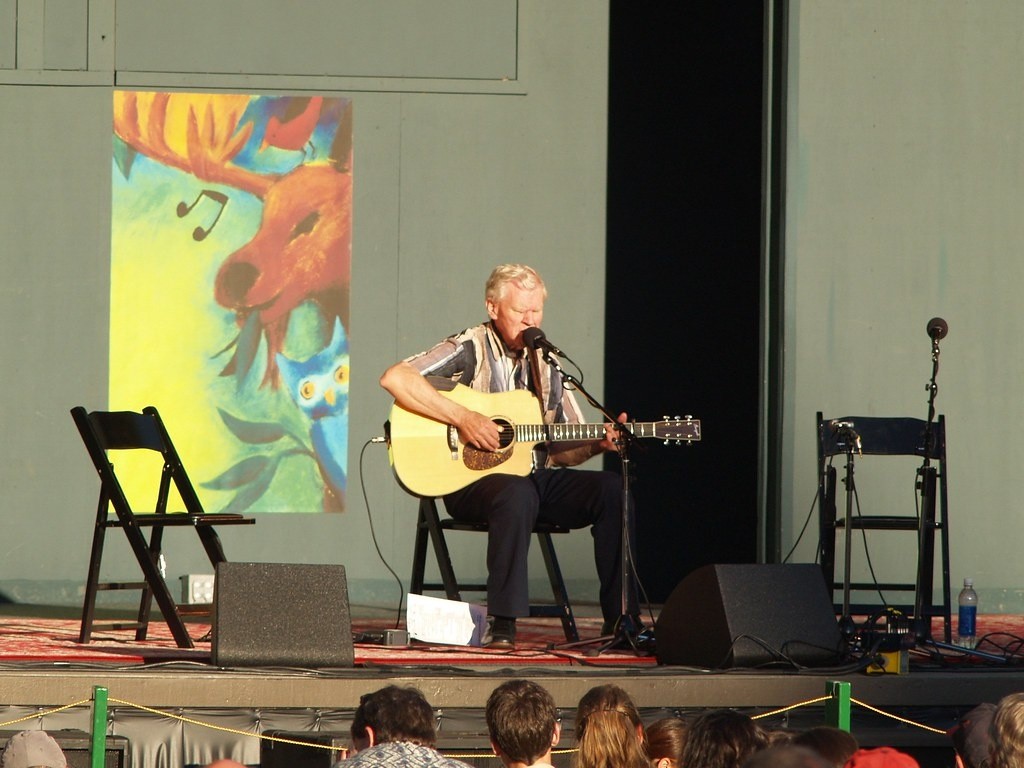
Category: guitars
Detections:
[{"x1": 385, "y1": 377, "x2": 703, "y2": 500}]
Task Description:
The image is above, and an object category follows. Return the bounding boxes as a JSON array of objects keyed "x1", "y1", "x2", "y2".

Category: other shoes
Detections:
[
  {"x1": 486, "y1": 615, "x2": 516, "y2": 648},
  {"x1": 601, "y1": 615, "x2": 656, "y2": 650}
]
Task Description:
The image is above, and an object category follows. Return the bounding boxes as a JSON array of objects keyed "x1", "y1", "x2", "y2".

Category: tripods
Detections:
[
  {"x1": 837, "y1": 333, "x2": 1021, "y2": 666},
  {"x1": 540, "y1": 340, "x2": 655, "y2": 658}
]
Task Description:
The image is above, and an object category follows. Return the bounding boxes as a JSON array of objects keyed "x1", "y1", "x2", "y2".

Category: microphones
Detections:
[
  {"x1": 829, "y1": 418, "x2": 859, "y2": 440},
  {"x1": 926, "y1": 317, "x2": 949, "y2": 339},
  {"x1": 523, "y1": 326, "x2": 566, "y2": 358}
]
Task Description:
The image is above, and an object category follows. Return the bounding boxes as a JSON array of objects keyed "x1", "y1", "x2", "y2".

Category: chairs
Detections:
[
  {"x1": 411, "y1": 496, "x2": 579, "y2": 643},
  {"x1": 815, "y1": 412, "x2": 951, "y2": 646},
  {"x1": 71, "y1": 406, "x2": 255, "y2": 651}
]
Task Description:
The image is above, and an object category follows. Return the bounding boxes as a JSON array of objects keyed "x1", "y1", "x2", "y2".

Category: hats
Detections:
[
  {"x1": 946, "y1": 703, "x2": 998, "y2": 768},
  {"x1": 844, "y1": 746, "x2": 920, "y2": 768},
  {"x1": 0, "y1": 731, "x2": 66, "y2": 768}
]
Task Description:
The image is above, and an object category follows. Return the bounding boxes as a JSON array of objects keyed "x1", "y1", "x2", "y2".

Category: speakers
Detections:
[
  {"x1": 212, "y1": 562, "x2": 354, "y2": 670},
  {"x1": 655, "y1": 563, "x2": 844, "y2": 668}
]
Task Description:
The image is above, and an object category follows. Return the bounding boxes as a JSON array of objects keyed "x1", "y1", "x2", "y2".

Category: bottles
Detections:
[{"x1": 959, "y1": 578, "x2": 978, "y2": 649}]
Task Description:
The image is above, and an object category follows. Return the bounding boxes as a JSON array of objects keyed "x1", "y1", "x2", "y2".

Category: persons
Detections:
[
  {"x1": 0, "y1": 676, "x2": 1024, "y2": 768},
  {"x1": 380, "y1": 263, "x2": 652, "y2": 652}
]
